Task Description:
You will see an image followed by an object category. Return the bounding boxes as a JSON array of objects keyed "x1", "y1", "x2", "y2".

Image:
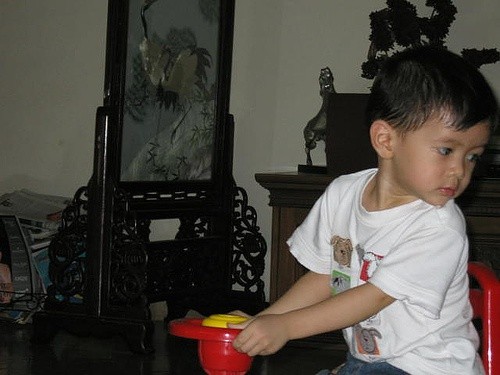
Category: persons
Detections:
[{"x1": 226, "y1": 46, "x2": 499, "y2": 375}]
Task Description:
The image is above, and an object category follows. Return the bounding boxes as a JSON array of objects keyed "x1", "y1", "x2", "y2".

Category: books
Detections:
[{"x1": 0, "y1": 189, "x2": 86, "y2": 327}]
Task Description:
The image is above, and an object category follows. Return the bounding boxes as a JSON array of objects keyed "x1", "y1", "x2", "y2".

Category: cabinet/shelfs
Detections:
[{"x1": 255, "y1": 168, "x2": 500, "y2": 375}]
